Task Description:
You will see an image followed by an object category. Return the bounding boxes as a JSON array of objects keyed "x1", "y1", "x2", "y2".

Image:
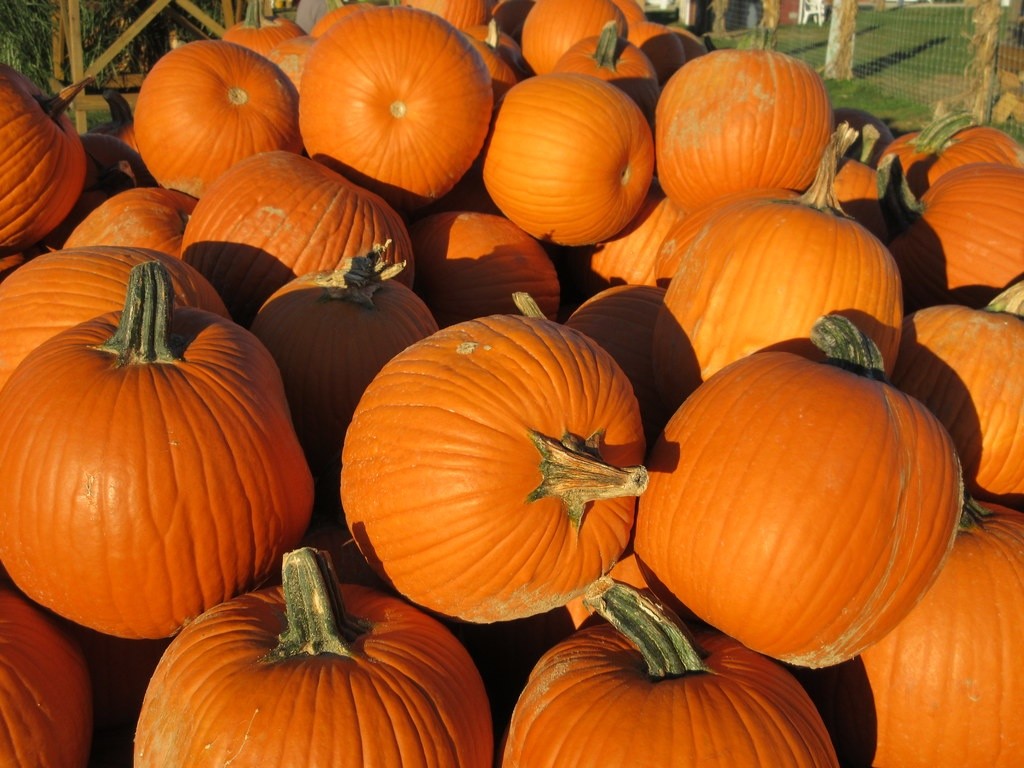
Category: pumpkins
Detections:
[{"x1": 0, "y1": 0, "x2": 1024, "y2": 767}]
